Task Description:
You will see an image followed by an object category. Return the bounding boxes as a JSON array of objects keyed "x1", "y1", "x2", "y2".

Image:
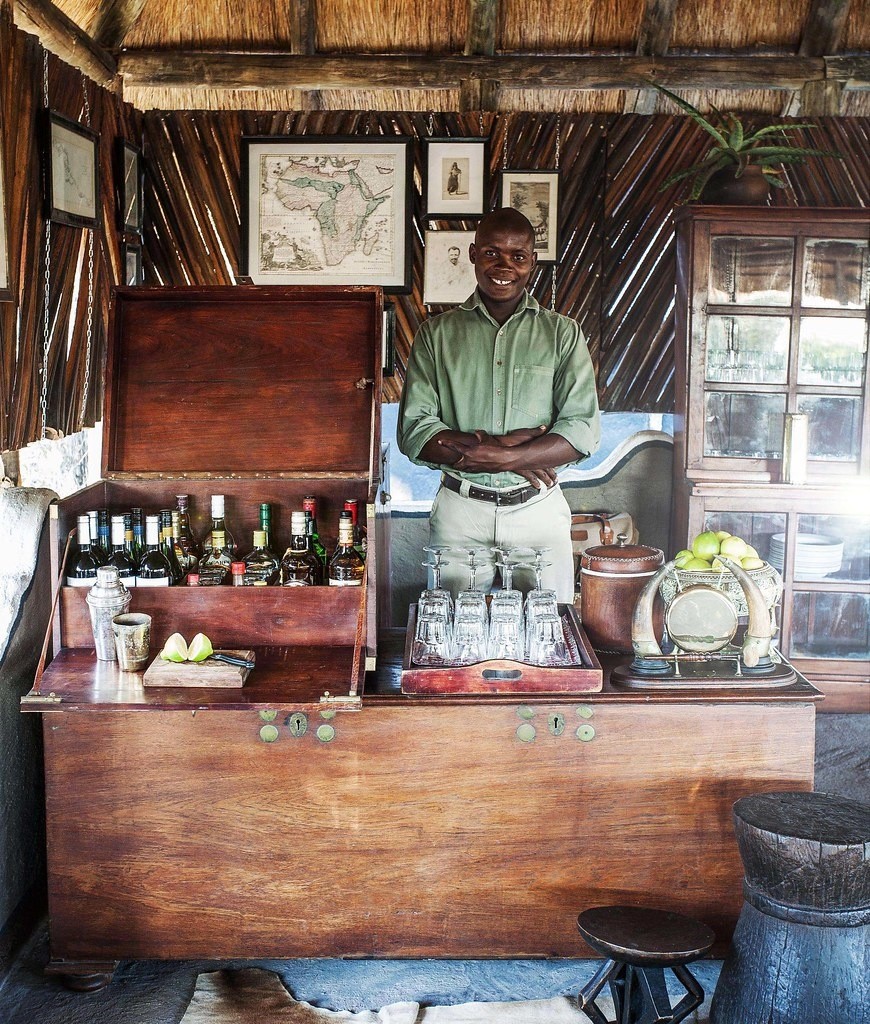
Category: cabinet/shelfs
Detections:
[
  {"x1": 671, "y1": 206, "x2": 870, "y2": 713},
  {"x1": 19, "y1": 280, "x2": 823, "y2": 962}
]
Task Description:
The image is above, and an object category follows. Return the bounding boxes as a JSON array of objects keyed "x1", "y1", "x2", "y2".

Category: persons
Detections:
[{"x1": 398, "y1": 208, "x2": 600, "y2": 603}]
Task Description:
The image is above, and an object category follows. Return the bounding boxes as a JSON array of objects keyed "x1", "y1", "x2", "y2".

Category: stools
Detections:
[{"x1": 576, "y1": 906, "x2": 715, "y2": 1024}]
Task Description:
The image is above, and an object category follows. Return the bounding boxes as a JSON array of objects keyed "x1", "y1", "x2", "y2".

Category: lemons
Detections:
[
  {"x1": 160, "y1": 632, "x2": 188, "y2": 662},
  {"x1": 672, "y1": 529, "x2": 763, "y2": 573},
  {"x1": 187, "y1": 632, "x2": 214, "y2": 662}
]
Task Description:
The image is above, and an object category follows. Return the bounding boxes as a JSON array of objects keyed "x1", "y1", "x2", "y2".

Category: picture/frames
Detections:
[
  {"x1": 424, "y1": 229, "x2": 479, "y2": 304},
  {"x1": 43, "y1": 106, "x2": 104, "y2": 228},
  {"x1": 420, "y1": 134, "x2": 492, "y2": 220},
  {"x1": 497, "y1": 168, "x2": 566, "y2": 265},
  {"x1": 113, "y1": 136, "x2": 144, "y2": 237},
  {"x1": 239, "y1": 135, "x2": 415, "y2": 296}
]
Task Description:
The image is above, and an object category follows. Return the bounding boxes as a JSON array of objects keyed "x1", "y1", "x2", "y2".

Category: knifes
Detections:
[{"x1": 208, "y1": 653, "x2": 255, "y2": 670}]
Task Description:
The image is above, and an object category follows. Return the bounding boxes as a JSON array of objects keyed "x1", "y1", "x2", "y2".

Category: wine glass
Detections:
[{"x1": 410, "y1": 545, "x2": 573, "y2": 668}]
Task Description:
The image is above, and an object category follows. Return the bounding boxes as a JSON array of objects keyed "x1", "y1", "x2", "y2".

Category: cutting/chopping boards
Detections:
[{"x1": 143, "y1": 649, "x2": 256, "y2": 688}]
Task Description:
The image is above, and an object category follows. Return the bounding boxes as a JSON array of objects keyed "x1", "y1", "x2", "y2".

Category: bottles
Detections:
[
  {"x1": 66, "y1": 494, "x2": 367, "y2": 585},
  {"x1": 84, "y1": 565, "x2": 133, "y2": 661}
]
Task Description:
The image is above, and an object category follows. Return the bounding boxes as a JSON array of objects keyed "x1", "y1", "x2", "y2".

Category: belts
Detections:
[{"x1": 440, "y1": 470, "x2": 561, "y2": 504}]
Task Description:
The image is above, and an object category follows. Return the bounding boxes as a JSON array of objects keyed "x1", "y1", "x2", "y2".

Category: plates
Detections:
[{"x1": 768, "y1": 532, "x2": 844, "y2": 580}]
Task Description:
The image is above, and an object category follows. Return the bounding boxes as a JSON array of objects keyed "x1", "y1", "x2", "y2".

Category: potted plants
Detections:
[{"x1": 639, "y1": 77, "x2": 850, "y2": 206}]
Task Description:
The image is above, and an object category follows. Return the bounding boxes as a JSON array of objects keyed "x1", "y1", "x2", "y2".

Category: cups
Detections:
[{"x1": 112, "y1": 612, "x2": 152, "y2": 672}]
